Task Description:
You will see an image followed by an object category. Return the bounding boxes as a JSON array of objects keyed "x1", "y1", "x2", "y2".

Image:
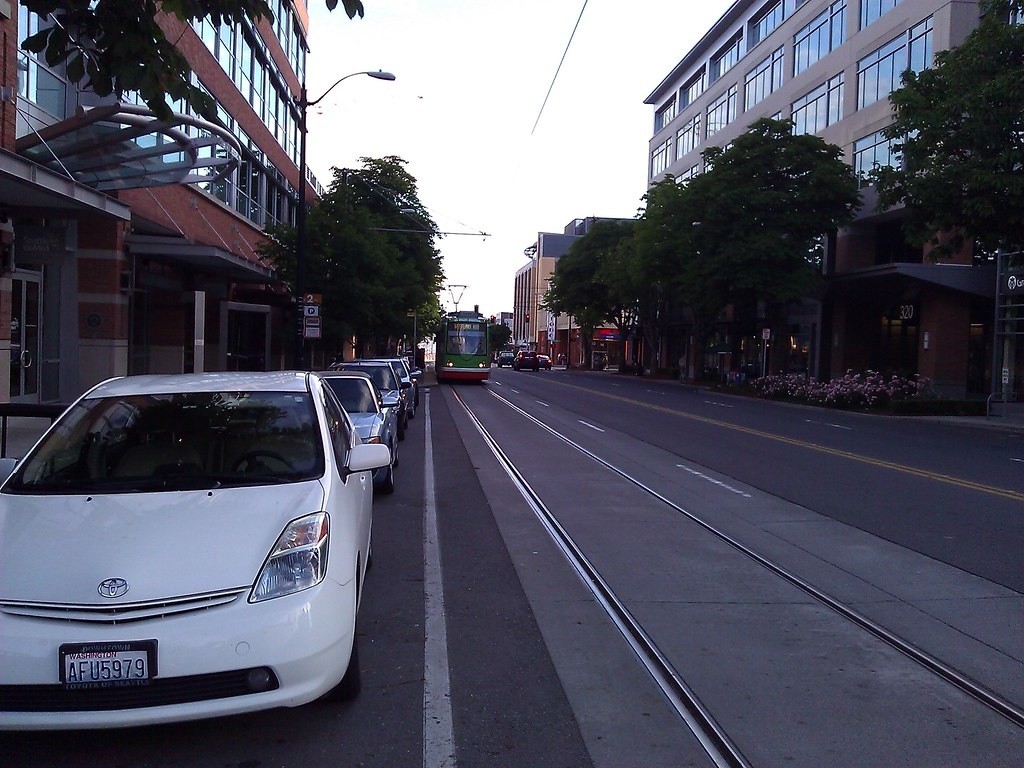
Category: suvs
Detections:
[
  {"x1": 512, "y1": 349, "x2": 540, "y2": 372},
  {"x1": 497, "y1": 351, "x2": 515, "y2": 368}
]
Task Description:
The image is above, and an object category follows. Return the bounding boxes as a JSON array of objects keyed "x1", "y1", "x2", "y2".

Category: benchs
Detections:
[{"x1": 135, "y1": 405, "x2": 313, "y2": 474}]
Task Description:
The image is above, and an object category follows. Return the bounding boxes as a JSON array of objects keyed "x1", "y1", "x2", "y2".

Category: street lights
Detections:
[{"x1": 290, "y1": 65, "x2": 398, "y2": 370}]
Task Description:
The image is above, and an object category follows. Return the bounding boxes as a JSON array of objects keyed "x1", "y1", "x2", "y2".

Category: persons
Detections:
[
  {"x1": 561, "y1": 352, "x2": 566, "y2": 366},
  {"x1": 602, "y1": 354, "x2": 608, "y2": 371}
]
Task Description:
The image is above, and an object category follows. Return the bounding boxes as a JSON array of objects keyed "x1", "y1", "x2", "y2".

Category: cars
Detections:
[
  {"x1": 538, "y1": 355, "x2": 553, "y2": 372},
  {"x1": 236, "y1": 371, "x2": 401, "y2": 495},
  {"x1": 326, "y1": 353, "x2": 423, "y2": 443},
  {"x1": 0, "y1": 367, "x2": 397, "y2": 735}
]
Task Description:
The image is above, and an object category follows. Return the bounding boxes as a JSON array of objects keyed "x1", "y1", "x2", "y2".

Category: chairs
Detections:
[
  {"x1": 115, "y1": 399, "x2": 203, "y2": 473},
  {"x1": 232, "y1": 395, "x2": 316, "y2": 471}
]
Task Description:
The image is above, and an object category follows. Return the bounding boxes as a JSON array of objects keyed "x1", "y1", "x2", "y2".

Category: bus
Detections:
[{"x1": 433, "y1": 284, "x2": 493, "y2": 384}]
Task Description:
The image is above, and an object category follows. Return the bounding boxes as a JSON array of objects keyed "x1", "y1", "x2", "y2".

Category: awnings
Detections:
[{"x1": 656, "y1": 304, "x2": 693, "y2": 328}]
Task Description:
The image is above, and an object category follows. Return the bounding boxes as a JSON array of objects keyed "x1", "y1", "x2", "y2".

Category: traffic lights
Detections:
[
  {"x1": 553, "y1": 344, "x2": 556, "y2": 348},
  {"x1": 491, "y1": 316, "x2": 494, "y2": 323},
  {"x1": 525, "y1": 313, "x2": 530, "y2": 323}
]
